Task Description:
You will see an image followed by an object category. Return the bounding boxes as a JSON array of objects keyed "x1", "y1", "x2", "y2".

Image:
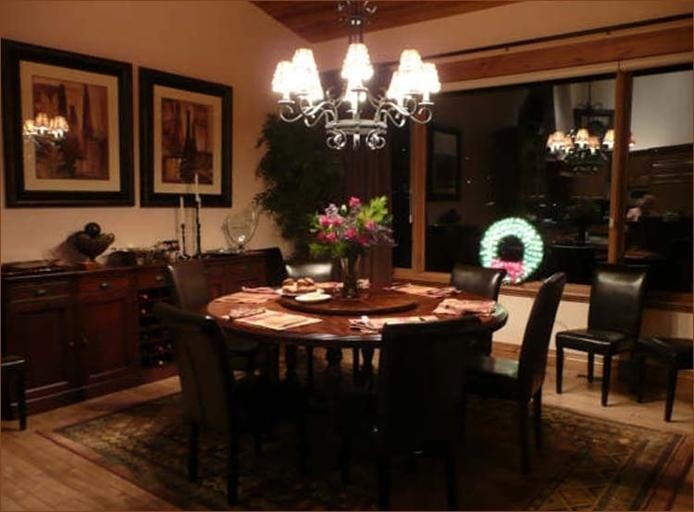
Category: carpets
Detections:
[{"x1": 35, "y1": 388, "x2": 692, "y2": 511}]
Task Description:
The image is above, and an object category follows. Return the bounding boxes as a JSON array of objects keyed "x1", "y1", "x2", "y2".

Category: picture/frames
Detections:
[
  {"x1": 426, "y1": 123, "x2": 463, "y2": 204},
  {"x1": 136, "y1": 64, "x2": 234, "y2": 211},
  {"x1": 0, "y1": 39, "x2": 136, "y2": 213},
  {"x1": 572, "y1": 107, "x2": 614, "y2": 137}
]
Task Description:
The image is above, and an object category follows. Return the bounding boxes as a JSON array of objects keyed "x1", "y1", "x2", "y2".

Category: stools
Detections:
[
  {"x1": 633, "y1": 335, "x2": 694, "y2": 422},
  {"x1": 0, "y1": 351, "x2": 29, "y2": 429}
]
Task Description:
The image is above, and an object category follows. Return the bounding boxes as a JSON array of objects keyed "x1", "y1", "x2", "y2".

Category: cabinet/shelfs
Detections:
[
  {"x1": 0, "y1": 269, "x2": 136, "y2": 420},
  {"x1": 202, "y1": 251, "x2": 281, "y2": 300},
  {"x1": 136, "y1": 266, "x2": 176, "y2": 384},
  {"x1": 424, "y1": 221, "x2": 479, "y2": 272}
]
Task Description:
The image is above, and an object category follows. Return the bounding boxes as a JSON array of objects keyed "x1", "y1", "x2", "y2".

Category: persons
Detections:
[{"x1": 626, "y1": 194, "x2": 660, "y2": 222}]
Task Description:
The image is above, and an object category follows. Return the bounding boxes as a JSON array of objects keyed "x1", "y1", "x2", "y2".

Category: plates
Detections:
[
  {"x1": 274, "y1": 283, "x2": 327, "y2": 298},
  {"x1": 294, "y1": 292, "x2": 334, "y2": 305}
]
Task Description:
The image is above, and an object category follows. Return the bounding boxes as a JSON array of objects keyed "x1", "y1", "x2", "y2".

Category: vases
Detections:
[
  {"x1": 576, "y1": 228, "x2": 588, "y2": 244},
  {"x1": 336, "y1": 251, "x2": 362, "y2": 298}
]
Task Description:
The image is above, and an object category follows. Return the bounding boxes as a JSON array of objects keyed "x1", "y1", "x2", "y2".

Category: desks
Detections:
[{"x1": 547, "y1": 240, "x2": 597, "y2": 282}]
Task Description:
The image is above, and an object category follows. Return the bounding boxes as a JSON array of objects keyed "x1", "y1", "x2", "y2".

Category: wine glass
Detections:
[{"x1": 73, "y1": 235, "x2": 115, "y2": 266}]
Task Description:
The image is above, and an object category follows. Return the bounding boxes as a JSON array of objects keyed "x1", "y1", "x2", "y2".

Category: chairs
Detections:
[
  {"x1": 552, "y1": 263, "x2": 648, "y2": 409},
  {"x1": 623, "y1": 213, "x2": 667, "y2": 271},
  {"x1": 151, "y1": 300, "x2": 312, "y2": 504},
  {"x1": 456, "y1": 272, "x2": 566, "y2": 479},
  {"x1": 161, "y1": 257, "x2": 279, "y2": 412},
  {"x1": 447, "y1": 264, "x2": 507, "y2": 358},
  {"x1": 338, "y1": 314, "x2": 481, "y2": 511},
  {"x1": 279, "y1": 258, "x2": 340, "y2": 379}
]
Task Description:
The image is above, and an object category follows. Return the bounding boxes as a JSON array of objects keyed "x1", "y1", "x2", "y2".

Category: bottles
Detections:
[
  {"x1": 54, "y1": 80, "x2": 94, "y2": 158},
  {"x1": 170, "y1": 103, "x2": 200, "y2": 180},
  {"x1": 163, "y1": 248, "x2": 176, "y2": 265}
]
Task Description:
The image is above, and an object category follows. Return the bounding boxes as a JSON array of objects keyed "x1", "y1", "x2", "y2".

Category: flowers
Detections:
[
  {"x1": 556, "y1": 199, "x2": 605, "y2": 228},
  {"x1": 305, "y1": 193, "x2": 400, "y2": 269}
]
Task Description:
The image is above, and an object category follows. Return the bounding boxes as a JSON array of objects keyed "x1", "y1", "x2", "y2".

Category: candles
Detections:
[
  {"x1": 178, "y1": 196, "x2": 185, "y2": 225},
  {"x1": 194, "y1": 172, "x2": 199, "y2": 201}
]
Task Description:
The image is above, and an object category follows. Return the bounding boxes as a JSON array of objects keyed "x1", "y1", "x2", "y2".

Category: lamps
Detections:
[
  {"x1": 22, "y1": 110, "x2": 69, "y2": 143},
  {"x1": 546, "y1": 78, "x2": 632, "y2": 181},
  {"x1": 266, "y1": 1, "x2": 441, "y2": 154}
]
